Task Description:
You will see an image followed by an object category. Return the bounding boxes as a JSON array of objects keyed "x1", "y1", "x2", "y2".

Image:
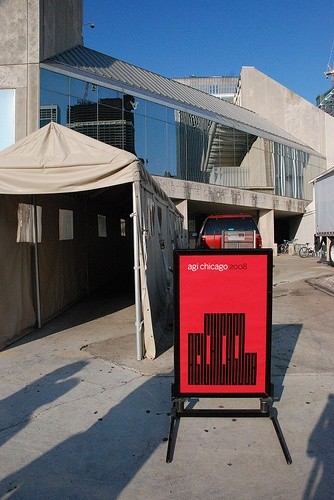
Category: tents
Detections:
[{"x1": 0, "y1": 120, "x2": 184, "y2": 362}]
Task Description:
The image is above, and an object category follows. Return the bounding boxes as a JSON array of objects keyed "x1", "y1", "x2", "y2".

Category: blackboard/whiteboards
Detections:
[{"x1": 169, "y1": 248, "x2": 274, "y2": 399}]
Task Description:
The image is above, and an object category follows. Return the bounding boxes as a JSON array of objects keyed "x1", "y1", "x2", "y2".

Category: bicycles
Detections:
[{"x1": 277, "y1": 239, "x2": 314, "y2": 258}]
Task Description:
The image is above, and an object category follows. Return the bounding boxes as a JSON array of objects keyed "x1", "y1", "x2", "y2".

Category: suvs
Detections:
[{"x1": 192, "y1": 214, "x2": 262, "y2": 248}]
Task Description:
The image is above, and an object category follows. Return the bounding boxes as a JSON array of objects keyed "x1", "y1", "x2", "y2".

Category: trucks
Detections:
[{"x1": 315, "y1": 172, "x2": 334, "y2": 267}]
